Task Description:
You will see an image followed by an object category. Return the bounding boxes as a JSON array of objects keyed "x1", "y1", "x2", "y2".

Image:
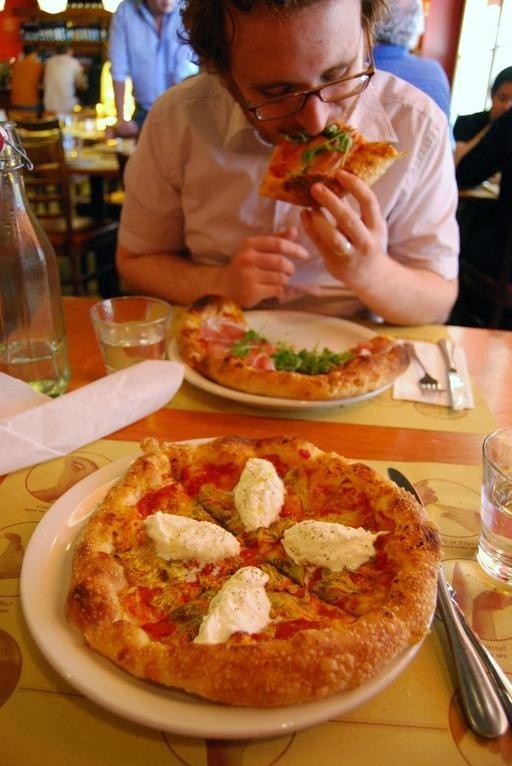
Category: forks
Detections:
[{"x1": 403, "y1": 341, "x2": 438, "y2": 398}]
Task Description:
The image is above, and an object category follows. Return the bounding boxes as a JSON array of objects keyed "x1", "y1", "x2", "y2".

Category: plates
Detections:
[
  {"x1": 167, "y1": 311, "x2": 410, "y2": 410},
  {"x1": 20, "y1": 436, "x2": 439, "y2": 740}
]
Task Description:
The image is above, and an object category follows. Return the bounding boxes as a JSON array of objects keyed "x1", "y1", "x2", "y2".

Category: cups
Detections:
[
  {"x1": 476, "y1": 427, "x2": 512, "y2": 584},
  {"x1": 90, "y1": 296, "x2": 173, "y2": 376}
]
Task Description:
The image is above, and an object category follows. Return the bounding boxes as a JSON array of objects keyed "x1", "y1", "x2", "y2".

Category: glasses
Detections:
[{"x1": 222, "y1": 16, "x2": 376, "y2": 122}]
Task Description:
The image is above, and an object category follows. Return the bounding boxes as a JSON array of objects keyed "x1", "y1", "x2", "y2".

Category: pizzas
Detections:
[
  {"x1": 176, "y1": 300, "x2": 410, "y2": 402},
  {"x1": 63, "y1": 431, "x2": 443, "y2": 708},
  {"x1": 259, "y1": 120, "x2": 407, "y2": 208}
]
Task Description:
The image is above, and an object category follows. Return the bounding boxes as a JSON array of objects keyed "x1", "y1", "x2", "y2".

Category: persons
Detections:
[
  {"x1": 444, "y1": 107, "x2": 512, "y2": 328},
  {"x1": 112, "y1": 0, "x2": 462, "y2": 329},
  {"x1": 108, "y1": 0, "x2": 205, "y2": 145},
  {"x1": 453, "y1": 67, "x2": 512, "y2": 184},
  {"x1": 42, "y1": 41, "x2": 90, "y2": 114},
  {"x1": 364, "y1": 0, "x2": 458, "y2": 155},
  {"x1": 9, "y1": 43, "x2": 43, "y2": 114}
]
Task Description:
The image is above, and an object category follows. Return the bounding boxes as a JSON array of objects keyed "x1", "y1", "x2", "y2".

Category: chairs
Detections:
[
  {"x1": 461, "y1": 226, "x2": 512, "y2": 328},
  {"x1": 13, "y1": 120, "x2": 121, "y2": 294}
]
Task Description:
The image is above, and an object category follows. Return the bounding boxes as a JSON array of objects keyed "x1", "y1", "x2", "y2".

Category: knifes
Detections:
[
  {"x1": 387, "y1": 465, "x2": 508, "y2": 737},
  {"x1": 437, "y1": 339, "x2": 467, "y2": 413}
]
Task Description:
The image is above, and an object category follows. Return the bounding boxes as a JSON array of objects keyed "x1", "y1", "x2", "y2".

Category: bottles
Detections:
[{"x1": 0, "y1": 111, "x2": 70, "y2": 399}]
